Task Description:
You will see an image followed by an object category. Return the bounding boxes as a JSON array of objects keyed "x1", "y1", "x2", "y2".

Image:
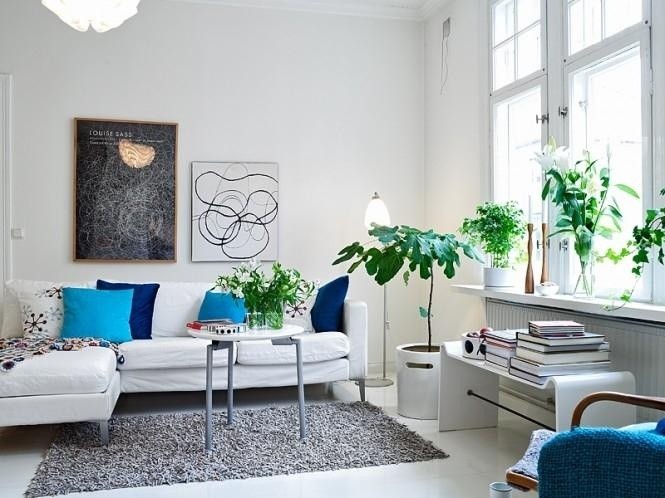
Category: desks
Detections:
[{"x1": 437, "y1": 339, "x2": 637, "y2": 439}]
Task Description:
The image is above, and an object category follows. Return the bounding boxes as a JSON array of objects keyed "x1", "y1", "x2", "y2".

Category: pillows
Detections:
[
  {"x1": 310, "y1": 272, "x2": 352, "y2": 334},
  {"x1": 7, "y1": 278, "x2": 89, "y2": 342},
  {"x1": 95, "y1": 279, "x2": 161, "y2": 339},
  {"x1": 197, "y1": 288, "x2": 248, "y2": 327},
  {"x1": 283, "y1": 278, "x2": 316, "y2": 336},
  {"x1": 58, "y1": 286, "x2": 136, "y2": 343}
]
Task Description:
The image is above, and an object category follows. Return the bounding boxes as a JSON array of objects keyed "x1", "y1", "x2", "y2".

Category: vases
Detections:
[
  {"x1": 242, "y1": 310, "x2": 284, "y2": 330},
  {"x1": 566, "y1": 230, "x2": 593, "y2": 296}
]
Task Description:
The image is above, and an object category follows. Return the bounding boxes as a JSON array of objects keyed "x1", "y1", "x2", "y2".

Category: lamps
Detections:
[
  {"x1": 41, "y1": 0, "x2": 141, "y2": 33},
  {"x1": 352, "y1": 191, "x2": 394, "y2": 390}
]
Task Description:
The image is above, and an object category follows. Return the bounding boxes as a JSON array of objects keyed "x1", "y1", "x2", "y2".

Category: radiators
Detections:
[{"x1": 484, "y1": 300, "x2": 664, "y2": 424}]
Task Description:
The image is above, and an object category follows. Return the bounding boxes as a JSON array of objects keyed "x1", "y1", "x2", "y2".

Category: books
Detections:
[
  {"x1": 188, "y1": 317, "x2": 234, "y2": 331},
  {"x1": 485, "y1": 316, "x2": 612, "y2": 385}
]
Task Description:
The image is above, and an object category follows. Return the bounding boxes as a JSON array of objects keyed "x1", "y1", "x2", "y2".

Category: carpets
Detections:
[{"x1": 23, "y1": 400, "x2": 453, "y2": 498}]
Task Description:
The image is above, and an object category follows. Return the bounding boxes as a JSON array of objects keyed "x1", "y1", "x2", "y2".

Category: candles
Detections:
[
  {"x1": 542, "y1": 191, "x2": 547, "y2": 223},
  {"x1": 528, "y1": 195, "x2": 533, "y2": 225}
]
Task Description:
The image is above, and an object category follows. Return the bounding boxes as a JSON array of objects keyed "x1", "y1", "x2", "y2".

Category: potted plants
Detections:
[
  {"x1": 329, "y1": 220, "x2": 483, "y2": 422},
  {"x1": 455, "y1": 196, "x2": 528, "y2": 287}
]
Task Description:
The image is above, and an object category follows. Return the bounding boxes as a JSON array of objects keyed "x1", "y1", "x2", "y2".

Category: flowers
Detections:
[
  {"x1": 530, "y1": 133, "x2": 641, "y2": 297},
  {"x1": 212, "y1": 255, "x2": 316, "y2": 330}
]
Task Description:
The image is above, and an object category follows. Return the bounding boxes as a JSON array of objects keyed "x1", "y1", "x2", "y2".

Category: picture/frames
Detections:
[
  {"x1": 72, "y1": 116, "x2": 181, "y2": 266},
  {"x1": 188, "y1": 159, "x2": 281, "y2": 262}
]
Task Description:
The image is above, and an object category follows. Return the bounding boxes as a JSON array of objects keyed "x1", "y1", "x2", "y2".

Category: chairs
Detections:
[{"x1": 505, "y1": 390, "x2": 664, "y2": 497}]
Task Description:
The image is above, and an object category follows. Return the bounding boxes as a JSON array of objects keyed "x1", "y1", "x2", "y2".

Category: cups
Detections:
[{"x1": 489, "y1": 481, "x2": 513, "y2": 498}]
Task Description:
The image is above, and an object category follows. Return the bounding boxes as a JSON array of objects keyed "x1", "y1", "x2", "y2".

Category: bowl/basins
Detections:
[{"x1": 536, "y1": 284, "x2": 560, "y2": 296}]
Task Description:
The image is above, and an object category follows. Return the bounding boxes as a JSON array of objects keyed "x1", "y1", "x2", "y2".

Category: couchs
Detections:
[{"x1": 0, "y1": 275, "x2": 371, "y2": 446}]
